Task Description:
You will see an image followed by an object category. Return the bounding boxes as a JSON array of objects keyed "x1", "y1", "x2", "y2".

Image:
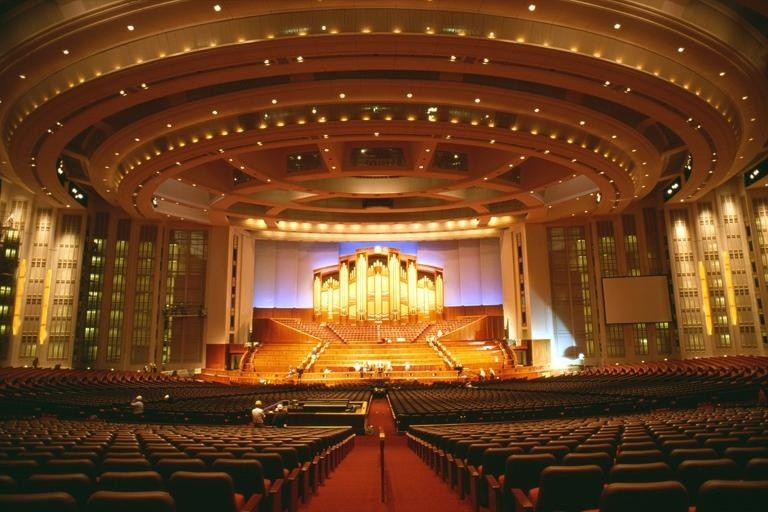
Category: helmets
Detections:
[
  {"x1": 255, "y1": 400, "x2": 262, "y2": 405},
  {"x1": 136, "y1": 396, "x2": 142, "y2": 400},
  {"x1": 277, "y1": 404, "x2": 283, "y2": 411}
]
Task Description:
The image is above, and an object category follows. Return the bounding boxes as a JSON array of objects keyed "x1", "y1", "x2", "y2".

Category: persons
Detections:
[
  {"x1": 252, "y1": 399, "x2": 266, "y2": 428},
  {"x1": 130, "y1": 395, "x2": 144, "y2": 420},
  {"x1": 312, "y1": 350, "x2": 317, "y2": 360},
  {"x1": 426, "y1": 330, "x2": 443, "y2": 345},
  {"x1": 354, "y1": 359, "x2": 392, "y2": 372},
  {"x1": 272, "y1": 404, "x2": 288, "y2": 428},
  {"x1": 480, "y1": 368, "x2": 485, "y2": 384},
  {"x1": 33, "y1": 358, "x2": 38, "y2": 368},
  {"x1": 489, "y1": 368, "x2": 495, "y2": 384}
]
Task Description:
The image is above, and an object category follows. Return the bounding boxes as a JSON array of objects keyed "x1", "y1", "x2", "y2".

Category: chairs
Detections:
[{"x1": 0, "y1": 345, "x2": 767, "y2": 510}]
